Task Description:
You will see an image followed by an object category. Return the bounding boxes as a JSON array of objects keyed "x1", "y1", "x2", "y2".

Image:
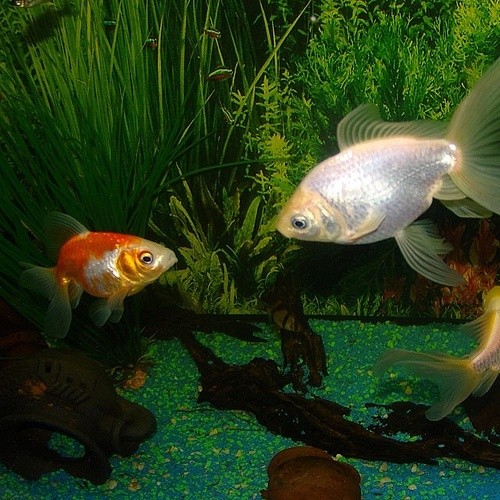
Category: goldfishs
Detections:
[
  {"x1": 17, "y1": 209, "x2": 178, "y2": 340},
  {"x1": 382, "y1": 284, "x2": 499, "y2": 422},
  {"x1": 273, "y1": 57, "x2": 499, "y2": 288}
]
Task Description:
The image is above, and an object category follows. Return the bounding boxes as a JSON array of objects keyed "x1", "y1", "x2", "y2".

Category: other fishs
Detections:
[
  {"x1": 260, "y1": 447, "x2": 362, "y2": 500},
  {"x1": 204, "y1": 68, "x2": 233, "y2": 84},
  {"x1": 145, "y1": 36, "x2": 157, "y2": 49},
  {"x1": 204, "y1": 27, "x2": 221, "y2": 39}
]
